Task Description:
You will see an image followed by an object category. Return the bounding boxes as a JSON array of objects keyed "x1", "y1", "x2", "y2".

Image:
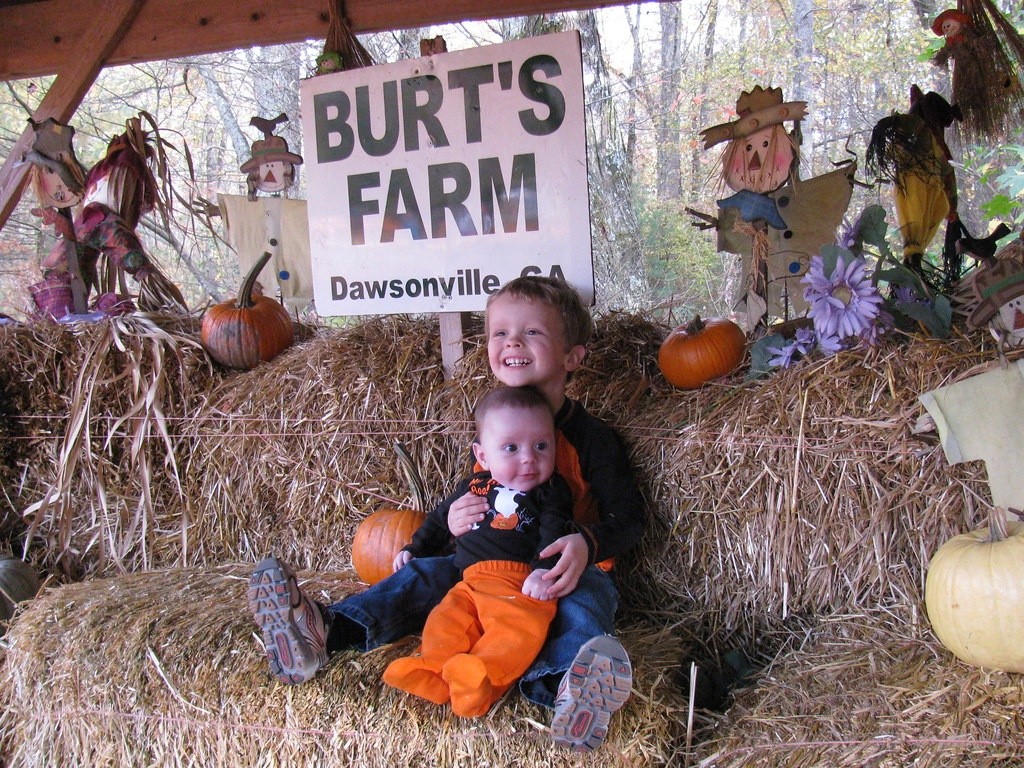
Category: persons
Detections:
[
  {"x1": 929, "y1": 8, "x2": 976, "y2": 66},
  {"x1": 316, "y1": 51, "x2": 342, "y2": 76},
  {"x1": 382, "y1": 388, "x2": 571, "y2": 719},
  {"x1": 248, "y1": 276, "x2": 633, "y2": 755}
]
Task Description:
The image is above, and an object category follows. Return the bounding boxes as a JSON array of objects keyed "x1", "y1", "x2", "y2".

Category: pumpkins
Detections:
[
  {"x1": 0, "y1": 554, "x2": 38, "y2": 638},
  {"x1": 352, "y1": 442, "x2": 429, "y2": 587},
  {"x1": 658, "y1": 314, "x2": 745, "y2": 389},
  {"x1": 925, "y1": 505, "x2": 1024, "y2": 676},
  {"x1": 201, "y1": 251, "x2": 294, "y2": 370}
]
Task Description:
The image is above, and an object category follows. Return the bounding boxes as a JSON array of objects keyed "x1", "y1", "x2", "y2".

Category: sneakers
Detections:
[
  {"x1": 250, "y1": 557, "x2": 336, "y2": 685},
  {"x1": 551, "y1": 635, "x2": 632, "y2": 751}
]
